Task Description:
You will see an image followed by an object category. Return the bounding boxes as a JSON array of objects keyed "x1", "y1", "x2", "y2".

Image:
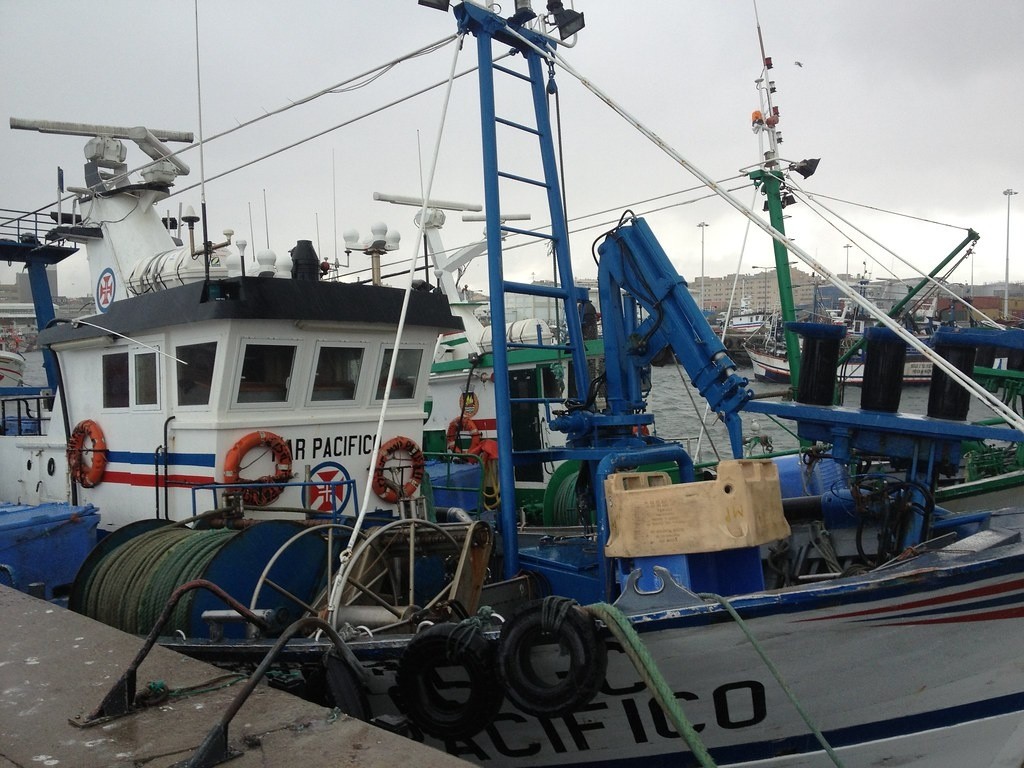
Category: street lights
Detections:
[
  {"x1": 970, "y1": 252, "x2": 976, "y2": 297},
  {"x1": 697, "y1": 222, "x2": 709, "y2": 309},
  {"x1": 1004, "y1": 189, "x2": 1018, "y2": 320},
  {"x1": 844, "y1": 244, "x2": 852, "y2": 285}
]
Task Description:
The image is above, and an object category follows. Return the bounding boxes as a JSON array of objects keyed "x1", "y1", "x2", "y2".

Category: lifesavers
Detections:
[
  {"x1": 495, "y1": 594, "x2": 611, "y2": 719},
  {"x1": 222, "y1": 430, "x2": 291, "y2": 506},
  {"x1": 446, "y1": 416, "x2": 480, "y2": 457},
  {"x1": 65, "y1": 419, "x2": 107, "y2": 488},
  {"x1": 394, "y1": 621, "x2": 507, "y2": 741},
  {"x1": 369, "y1": 436, "x2": 424, "y2": 502}
]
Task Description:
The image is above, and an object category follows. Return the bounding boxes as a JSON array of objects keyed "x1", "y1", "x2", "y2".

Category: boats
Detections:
[
  {"x1": 716, "y1": 276, "x2": 1024, "y2": 384},
  {"x1": 0, "y1": 0, "x2": 1024, "y2": 768}
]
